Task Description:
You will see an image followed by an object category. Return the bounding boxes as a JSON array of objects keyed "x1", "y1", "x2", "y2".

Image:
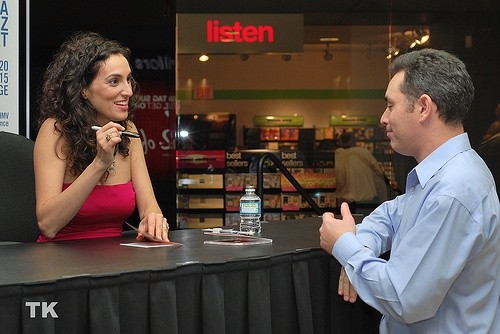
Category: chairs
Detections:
[{"x1": 0, "y1": 131, "x2": 39, "y2": 245}]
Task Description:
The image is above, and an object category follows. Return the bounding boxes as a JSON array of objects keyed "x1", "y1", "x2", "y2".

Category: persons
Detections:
[
  {"x1": 33, "y1": 32, "x2": 169, "y2": 243},
  {"x1": 319, "y1": 47, "x2": 500, "y2": 334},
  {"x1": 477, "y1": 104, "x2": 500, "y2": 202},
  {"x1": 335, "y1": 134, "x2": 388, "y2": 215}
]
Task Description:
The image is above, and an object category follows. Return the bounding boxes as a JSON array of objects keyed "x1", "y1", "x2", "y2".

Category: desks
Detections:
[{"x1": 0, "y1": 214, "x2": 391, "y2": 334}]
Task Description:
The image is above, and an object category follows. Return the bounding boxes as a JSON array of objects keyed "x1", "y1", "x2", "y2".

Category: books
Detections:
[
  {"x1": 120, "y1": 222, "x2": 183, "y2": 248},
  {"x1": 203, "y1": 233, "x2": 273, "y2": 245}
]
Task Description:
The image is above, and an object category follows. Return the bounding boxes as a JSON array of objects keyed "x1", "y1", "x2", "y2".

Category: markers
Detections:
[{"x1": 91, "y1": 125, "x2": 141, "y2": 138}]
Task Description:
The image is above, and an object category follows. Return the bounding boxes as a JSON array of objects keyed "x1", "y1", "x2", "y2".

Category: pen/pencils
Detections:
[{"x1": 203, "y1": 227, "x2": 241, "y2": 233}]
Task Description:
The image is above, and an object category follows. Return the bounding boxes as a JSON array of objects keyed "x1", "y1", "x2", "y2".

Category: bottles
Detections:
[{"x1": 240, "y1": 185, "x2": 261, "y2": 235}]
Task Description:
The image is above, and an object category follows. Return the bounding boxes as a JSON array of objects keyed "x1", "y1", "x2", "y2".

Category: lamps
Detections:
[
  {"x1": 282, "y1": 55, "x2": 291, "y2": 61},
  {"x1": 240, "y1": 54, "x2": 249, "y2": 61},
  {"x1": 324, "y1": 48, "x2": 333, "y2": 61}
]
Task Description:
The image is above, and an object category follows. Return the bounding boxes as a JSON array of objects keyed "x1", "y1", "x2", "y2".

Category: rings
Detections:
[
  {"x1": 106, "y1": 133, "x2": 111, "y2": 143},
  {"x1": 163, "y1": 225, "x2": 168, "y2": 229}
]
